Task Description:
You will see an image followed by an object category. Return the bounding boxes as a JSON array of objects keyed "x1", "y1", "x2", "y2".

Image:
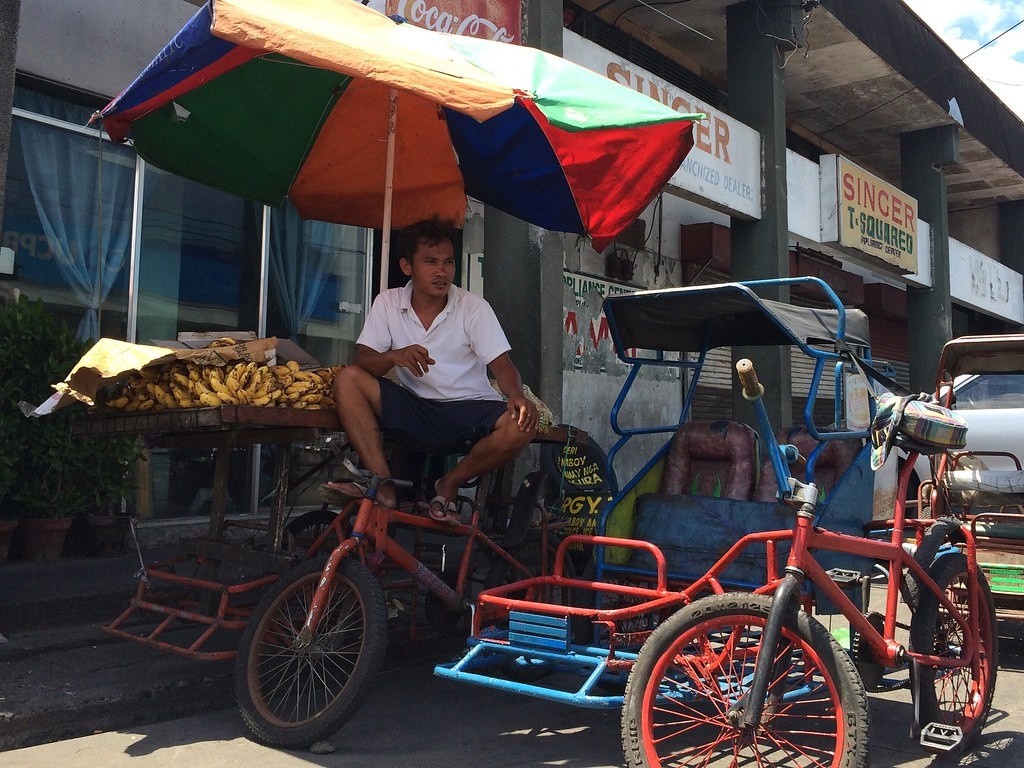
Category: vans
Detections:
[{"x1": 897, "y1": 334, "x2": 1024, "y2": 520}]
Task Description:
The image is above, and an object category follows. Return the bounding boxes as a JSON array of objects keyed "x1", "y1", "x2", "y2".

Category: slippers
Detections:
[
  {"x1": 424, "y1": 478, "x2": 456, "y2": 520},
  {"x1": 317, "y1": 481, "x2": 396, "y2": 509}
]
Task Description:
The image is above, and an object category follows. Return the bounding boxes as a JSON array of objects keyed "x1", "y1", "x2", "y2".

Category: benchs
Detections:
[{"x1": 943, "y1": 470, "x2": 1024, "y2": 539}]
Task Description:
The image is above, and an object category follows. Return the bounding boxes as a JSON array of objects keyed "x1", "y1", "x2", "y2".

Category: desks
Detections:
[{"x1": 73, "y1": 405, "x2": 587, "y2": 617}]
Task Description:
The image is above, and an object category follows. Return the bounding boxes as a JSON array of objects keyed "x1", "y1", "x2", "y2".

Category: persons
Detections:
[{"x1": 335, "y1": 220, "x2": 539, "y2": 521}]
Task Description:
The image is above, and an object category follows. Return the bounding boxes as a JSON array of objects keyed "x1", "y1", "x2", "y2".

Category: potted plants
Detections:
[
  {"x1": 0, "y1": 293, "x2": 45, "y2": 563},
  {"x1": 81, "y1": 433, "x2": 148, "y2": 558},
  {"x1": 18, "y1": 320, "x2": 95, "y2": 562}
]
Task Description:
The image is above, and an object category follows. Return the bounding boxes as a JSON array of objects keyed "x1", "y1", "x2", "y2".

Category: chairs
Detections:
[
  {"x1": 658, "y1": 421, "x2": 760, "y2": 501},
  {"x1": 755, "y1": 427, "x2": 863, "y2": 502}
]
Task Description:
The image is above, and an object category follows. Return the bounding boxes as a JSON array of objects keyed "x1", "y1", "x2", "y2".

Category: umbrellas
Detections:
[{"x1": 89, "y1": 0, "x2": 709, "y2": 295}]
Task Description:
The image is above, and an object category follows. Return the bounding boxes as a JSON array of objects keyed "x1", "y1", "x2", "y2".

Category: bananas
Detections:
[{"x1": 103, "y1": 335, "x2": 349, "y2": 411}]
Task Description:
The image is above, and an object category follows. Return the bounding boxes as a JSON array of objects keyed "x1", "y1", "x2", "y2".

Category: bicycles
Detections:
[{"x1": 232, "y1": 275, "x2": 1023, "y2": 768}]
